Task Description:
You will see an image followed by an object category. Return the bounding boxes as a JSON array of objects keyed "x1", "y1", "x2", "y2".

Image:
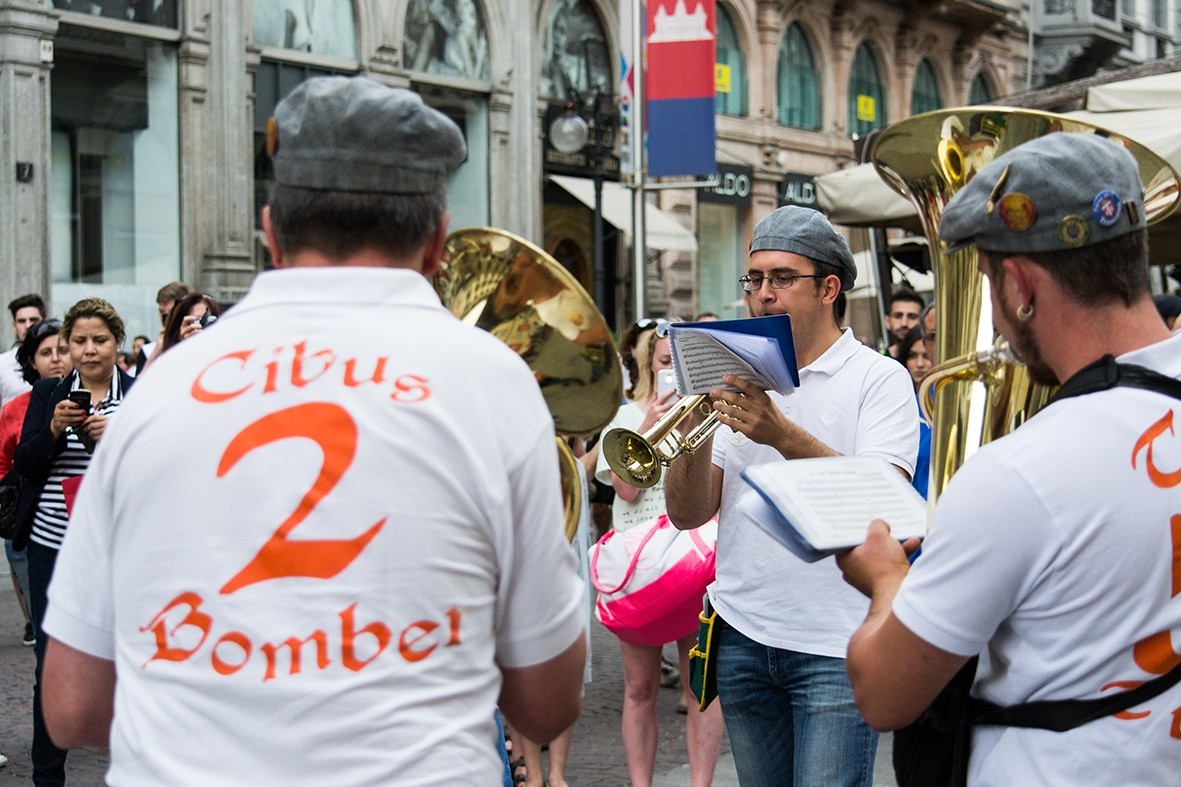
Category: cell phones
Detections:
[
  {"x1": 69, "y1": 389, "x2": 91, "y2": 425},
  {"x1": 659, "y1": 369, "x2": 678, "y2": 405}
]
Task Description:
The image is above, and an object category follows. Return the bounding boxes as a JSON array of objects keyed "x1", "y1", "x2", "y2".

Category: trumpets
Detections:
[{"x1": 600, "y1": 385, "x2": 750, "y2": 486}]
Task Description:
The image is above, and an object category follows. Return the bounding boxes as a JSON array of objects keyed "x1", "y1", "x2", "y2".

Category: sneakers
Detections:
[{"x1": 22, "y1": 623, "x2": 37, "y2": 646}]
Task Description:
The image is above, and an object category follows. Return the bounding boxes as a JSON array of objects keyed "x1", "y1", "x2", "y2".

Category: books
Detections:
[
  {"x1": 736, "y1": 456, "x2": 928, "y2": 564},
  {"x1": 665, "y1": 315, "x2": 799, "y2": 396}
]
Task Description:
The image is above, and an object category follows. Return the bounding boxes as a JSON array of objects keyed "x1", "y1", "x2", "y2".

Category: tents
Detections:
[{"x1": 812, "y1": 70, "x2": 1181, "y2": 348}]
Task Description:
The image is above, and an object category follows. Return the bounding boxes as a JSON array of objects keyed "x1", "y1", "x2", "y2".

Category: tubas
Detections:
[
  {"x1": 870, "y1": 102, "x2": 1180, "y2": 499},
  {"x1": 436, "y1": 224, "x2": 622, "y2": 547}
]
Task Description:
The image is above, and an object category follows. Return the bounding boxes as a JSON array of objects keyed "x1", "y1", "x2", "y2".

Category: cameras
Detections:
[{"x1": 189, "y1": 307, "x2": 217, "y2": 329}]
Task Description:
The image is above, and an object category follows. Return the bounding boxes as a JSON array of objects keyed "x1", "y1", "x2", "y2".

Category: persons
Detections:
[
  {"x1": 0, "y1": 283, "x2": 1181, "y2": 787},
  {"x1": 41, "y1": 75, "x2": 586, "y2": 787},
  {"x1": 666, "y1": 204, "x2": 920, "y2": 787},
  {"x1": 838, "y1": 132, "x2": 1181, "y2": 787}
]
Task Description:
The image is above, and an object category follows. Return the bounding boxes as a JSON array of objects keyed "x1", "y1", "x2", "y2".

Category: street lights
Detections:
[{"x1": 545, "y1": 82, "x2": 623, "y2": 314}]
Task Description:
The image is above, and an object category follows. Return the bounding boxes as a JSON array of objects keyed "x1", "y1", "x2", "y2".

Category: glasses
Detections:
[
  {"x1": 175, "y1": 292, "x2": 214, "y2": 305},
  {"x1": 637, "y1": 318, "x2": 666, "y2": 328},
  {"x1": 739, "y1": 273, "x2": 826, "y2": 291},
  {"x1": 654, "y1": 321, "x2": 672, "y2": 338},
  {"x1": 923, "y1": 331, "x2": 935, "y2": 344},
  {"x1": 36, "y1": 320, "x2": 64, "y2": 338}
]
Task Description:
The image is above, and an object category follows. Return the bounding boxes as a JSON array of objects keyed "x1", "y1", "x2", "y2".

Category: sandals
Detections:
[
  {"x1": 511, "y1": 759, "x2": 526, "y2": 787},
  {"x1": 503, "y1": 725, "x2": 513, "y2": 754}
]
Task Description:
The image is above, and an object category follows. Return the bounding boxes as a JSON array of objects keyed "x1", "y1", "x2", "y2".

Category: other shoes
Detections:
[
  {"x1": 676, "y1": 685, "x2": 687, "y2": 713},
  {"x1": 0, "y1": 752, "x2": 9, "y2": 767},
  {"x1": 658, "y1": 655, "x2": 681, "y2": 687}
]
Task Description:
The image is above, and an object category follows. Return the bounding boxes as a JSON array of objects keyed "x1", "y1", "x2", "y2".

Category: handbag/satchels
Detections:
[
  {"x1": 688, "y1": 612, "x2": 720, "y2": 712},
  {"x1": 0, "y1": 468, "x2": 26, "y2": 539},
  {"x1": 588, "y1": 513, "x2": 720, "y2": 647},
  {"x1": 891, "y1": 654, "x2": 978, "y2": 787}
]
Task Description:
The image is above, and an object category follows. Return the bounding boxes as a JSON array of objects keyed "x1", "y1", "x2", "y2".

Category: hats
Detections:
[
  {"x1": 937, "y1": 131, "x2": 1146, "y2": 254},
  {"x1": 265, "y1": 76, "x2": 467, "y2": 194},
  {"x1": 749, "y1": 204, "x2": 857, "y2": 291}
]
